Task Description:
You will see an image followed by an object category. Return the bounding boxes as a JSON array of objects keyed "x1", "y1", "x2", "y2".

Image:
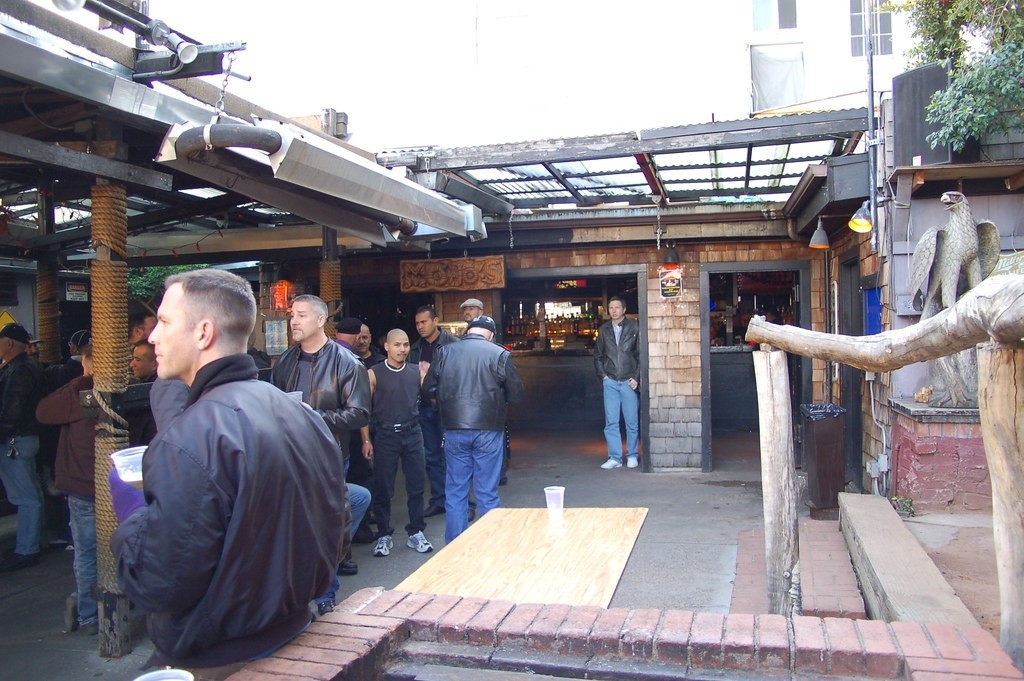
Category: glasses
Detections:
[{"x1": 68, "y1": 342, "x2": 71, "y2": 347}]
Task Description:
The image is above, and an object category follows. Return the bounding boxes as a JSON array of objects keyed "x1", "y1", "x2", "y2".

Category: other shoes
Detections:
[
  {"x1": 338, "y1": 559, "x2": 358, "y2": 572},
  {"x1": 80, "y1": 622, "x2": 99, "y2": 636},
  {"x1": 64, "y1": 596, "x2": 78, "y2": 632},
  {"x1": 0, "y1": 551, "x2": 42, "y2": 571},
  {"x1": 499, "y1": 477, "x2": 508, "y2": 485}
]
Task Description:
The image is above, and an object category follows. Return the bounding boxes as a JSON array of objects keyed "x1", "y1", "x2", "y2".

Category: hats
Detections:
[
  {"x1": 71, "y1": 330, "x2": 92, "y2": 348},
  {"x1": 460, "y1": 298, "x2": 483, "y2": 310},
  {"x1": 335, "y1": 318, "x2": 362, "y2": 334},
  {"x1": 0, "y1": 324, "x2": 30, "y2": 344},
  {"x1": 466, "y1": 315, "x2": 496, "y2": 338}
]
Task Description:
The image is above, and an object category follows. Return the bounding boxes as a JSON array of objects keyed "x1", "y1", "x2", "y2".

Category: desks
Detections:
[{"x1": 395, "y1": 507, "x2": 649, "y2": 609}]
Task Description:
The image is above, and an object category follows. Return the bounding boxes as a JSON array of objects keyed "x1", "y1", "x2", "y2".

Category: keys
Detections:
[{"x1": 6, "y1": 447, "x2": 19, "y2": 460}]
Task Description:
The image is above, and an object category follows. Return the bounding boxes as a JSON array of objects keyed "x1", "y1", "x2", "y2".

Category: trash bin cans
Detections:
[{"x1": 801, "y1": 402, "x2": 846, "y2": 509}]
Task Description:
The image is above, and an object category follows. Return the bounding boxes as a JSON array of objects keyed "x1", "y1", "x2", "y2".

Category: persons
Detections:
[
  {"x1": 594, "y1": 297, "x2": 638, "y2": 468},
  {"x1": 420, "y1": 315, "x2": 526, "y2": 546},
  {"x1": 338, "y1": 483, "x2": 371, "y2": 576},
  {"x1": 334, "y1": 317, "x2": 387, "y2": 542},
  {"x1": 408, "y1": 308, "x2": 475, "y2": 522},
  {"x1": 109, "y1": 268, "x2": 349, "y2": 680},
  {"x1": 359, "y1": 328, "x2": 433, "y2": 556},
  {"x1": 0, "y1": 323, "x2": 47, "y2": 571},
  {"x1": 36, "y1": 311, "x2": 191, "y2": 636},
  {"x1": 248, "y1": 330, "x2": 270, "y2": 382},
  {"x1": 460, "y1": 298, "x2": 512, "y2": 484},
  {"x1": 270, "y1": 294, "x2": 371, "y2": 617}
]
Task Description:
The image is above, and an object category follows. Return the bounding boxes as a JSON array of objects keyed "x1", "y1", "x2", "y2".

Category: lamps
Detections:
[
  {"x1": 848, "y1": 197, "x2": 893, "y2": 233},
  {"x1": 152, "y1": 23, "x2": 198, "y2": 64},
  {"x1": 808, "y1": 214, "x2": 853, "y2": 249},
  {"x1": 663, "y1": 240, "x2": 678, "y2": 263}
]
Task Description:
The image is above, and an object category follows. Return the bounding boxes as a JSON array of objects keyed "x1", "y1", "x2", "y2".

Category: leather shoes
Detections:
[
  {"x1": 467, "y1": 509, "x2": 475, "y2": 522},
  {"x1": 424, "y1": 506, "x2": 445, "y2": 517}
]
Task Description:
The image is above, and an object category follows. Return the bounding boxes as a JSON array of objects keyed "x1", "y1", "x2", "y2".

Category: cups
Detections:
[
  {"x1": 542, "y1": 485, "x2": 566, "y2": 518},
  {"x1": 286, "y1": 390, "x2": 303, "y2": 402},
  {"x1": 110, "y1": 445, "x2": 149, "y2": 492},
  {"x1": 133, "y1": 669, "x2": 195, "y2": 681}
]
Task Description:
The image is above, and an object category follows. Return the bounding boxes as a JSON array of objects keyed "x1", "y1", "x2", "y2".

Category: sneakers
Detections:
[
  {"x1": 317, "y1": 600, "x2": 336, "y2": 616},
  {"x1": 406, "y1": 530, "x2": 433, "y2": 553},
  {"x1": 371, "y1": 535, "x2": 393, "y2": 556},
  {"x1": 601, "y1": 458, "x2": 622, "y2": 469},
  {"x1": 627, "y1": 457, "x2": 638, "y2": 468}
]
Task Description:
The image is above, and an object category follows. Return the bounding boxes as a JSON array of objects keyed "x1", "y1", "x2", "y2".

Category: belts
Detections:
[{"x1": 380, "y1": 420, "x2": 417, "y2": 433}]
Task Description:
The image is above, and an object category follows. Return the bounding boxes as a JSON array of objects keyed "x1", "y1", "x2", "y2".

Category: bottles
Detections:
[
  {"x1": 504, "y1": 311, "x2": 605, "y2": 350},
  {"x1": 710, "y1": 304, "x2": 794, "y2": 346}
]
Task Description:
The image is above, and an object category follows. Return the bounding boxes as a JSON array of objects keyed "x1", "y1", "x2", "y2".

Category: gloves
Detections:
[{"x1": 108, "y1": 470, "x2": 149, "y2": 522}]
